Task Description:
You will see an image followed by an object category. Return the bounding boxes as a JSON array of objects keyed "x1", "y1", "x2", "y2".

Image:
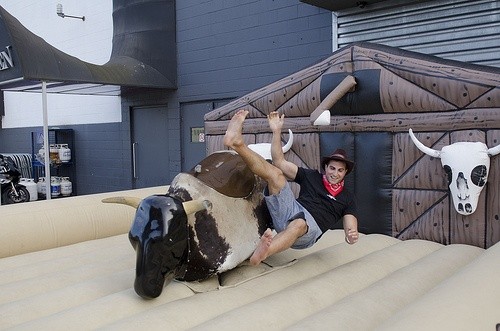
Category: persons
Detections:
[{"x1": 225, "y1": 110, "x2": 359, "y2": 266}]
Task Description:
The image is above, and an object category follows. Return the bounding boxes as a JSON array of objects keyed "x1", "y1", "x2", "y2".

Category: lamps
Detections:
[{"x1": 56, "y1": 0, "x2": 86, "y2": 21}]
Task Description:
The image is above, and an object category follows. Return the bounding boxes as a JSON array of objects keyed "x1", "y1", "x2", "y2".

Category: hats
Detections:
[{"x1": 322, "y1": 149, "x2": 354, "y2": 174}]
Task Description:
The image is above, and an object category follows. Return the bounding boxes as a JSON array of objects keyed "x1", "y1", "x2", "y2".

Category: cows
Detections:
[{"x1": 101, "y1": 148, "x2": 277, "y2": 301}]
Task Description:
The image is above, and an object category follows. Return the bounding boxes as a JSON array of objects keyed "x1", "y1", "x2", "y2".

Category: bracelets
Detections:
[{"x1": 347, "y1": 237, "x2": 357, "y2": 244}]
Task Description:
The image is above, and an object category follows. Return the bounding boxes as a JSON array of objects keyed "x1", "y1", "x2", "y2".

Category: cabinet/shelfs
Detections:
[{"x1": 31, "y1": 127, "x2": 77, "y2": 198}]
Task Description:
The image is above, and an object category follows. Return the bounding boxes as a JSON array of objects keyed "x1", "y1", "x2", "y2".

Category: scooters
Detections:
[{"x1": 0, "y1": 153, "x2": 31, "y2": 205}]
